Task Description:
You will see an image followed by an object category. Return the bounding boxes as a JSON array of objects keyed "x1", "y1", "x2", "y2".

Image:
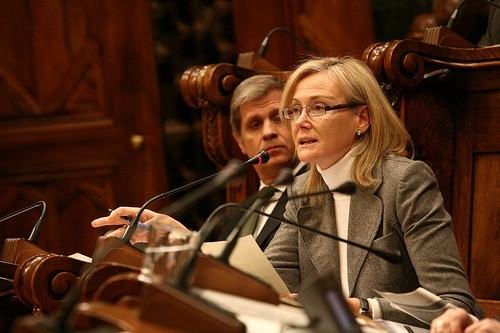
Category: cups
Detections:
[{"x1": 140, "y1": 224, "x2": 200, "y2": 285}]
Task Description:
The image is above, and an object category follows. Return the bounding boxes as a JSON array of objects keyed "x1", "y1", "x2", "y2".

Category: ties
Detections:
[{"x1": 240, "y1": 186, "x2": 280, "y2": 238}]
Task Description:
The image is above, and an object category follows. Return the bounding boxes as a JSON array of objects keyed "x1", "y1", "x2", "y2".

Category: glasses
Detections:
[{"x1": 281, "y1": 101, "x2": 367, "y2": 119}]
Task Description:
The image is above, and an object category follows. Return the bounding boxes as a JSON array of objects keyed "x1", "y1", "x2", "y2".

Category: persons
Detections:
[{"x1": 91, "y1": 55, "x2": 500, "y2": 333}]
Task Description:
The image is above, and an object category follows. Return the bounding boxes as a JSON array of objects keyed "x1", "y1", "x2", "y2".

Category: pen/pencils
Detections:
[{"x1": 108, "y1": 209, "x2": 149, "y2": 231}]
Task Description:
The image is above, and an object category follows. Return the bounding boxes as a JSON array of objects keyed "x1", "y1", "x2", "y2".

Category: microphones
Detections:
[
  {"x1": 122, "y1": 150, "x2": 270, "y2": 241},
  {"x1": 159, "y1": 168, "x2": 402, "y2": 291}
]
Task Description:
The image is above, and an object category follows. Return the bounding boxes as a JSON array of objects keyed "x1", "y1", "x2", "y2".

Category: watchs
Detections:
[{"x1": 358, "y1": 297, "x2": 374, "y2": 318}]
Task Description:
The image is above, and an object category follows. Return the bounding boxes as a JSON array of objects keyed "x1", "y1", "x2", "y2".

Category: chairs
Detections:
[{"x1": 180, "y1": 26, "x2": 500, "y2": 321}]
